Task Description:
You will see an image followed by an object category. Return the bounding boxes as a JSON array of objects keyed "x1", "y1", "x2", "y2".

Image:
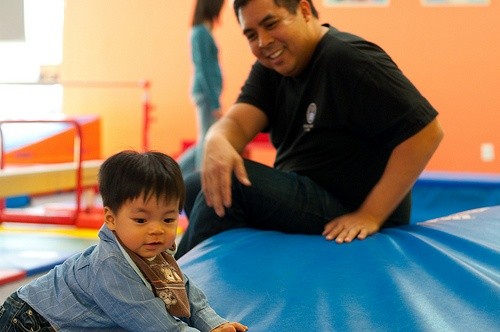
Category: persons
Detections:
[
  {"x1": 174, "y1": 0, "x2": 224, "y2": 179},
  {"x1": 171, "y1": 0, "x2": 443, "y2": 261},
  {"x1": 0, "y1": 149, "x2": 249, "y2": 332}
]
